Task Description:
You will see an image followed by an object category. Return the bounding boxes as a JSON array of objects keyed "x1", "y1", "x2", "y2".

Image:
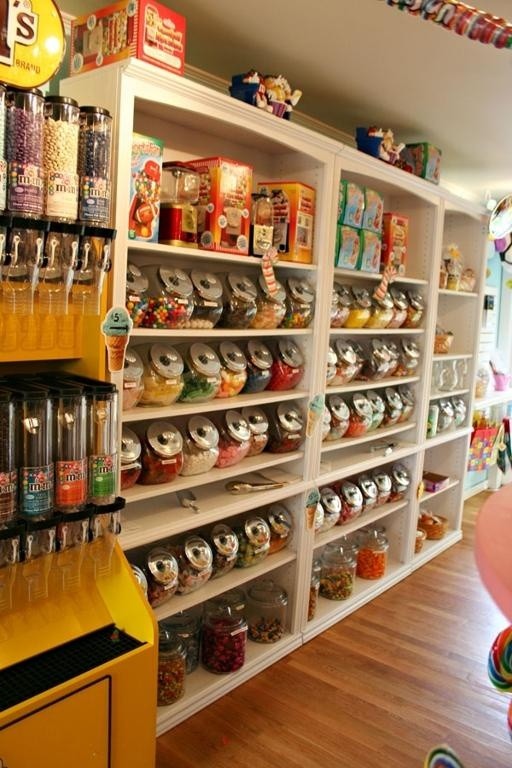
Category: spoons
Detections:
[{"x1": 177, "y1": 489, "x2": 201, "y2": 515}]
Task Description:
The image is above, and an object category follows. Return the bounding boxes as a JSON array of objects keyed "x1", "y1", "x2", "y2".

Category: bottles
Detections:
[
  {"x1": 241, "y1": 408, "x2": 268, "y2": 457},
  {"x1": 140, "y1": 342, "x2": 183, "y2": 407},
  {"x1": 386, "y1": 287, "x2": 406, "y2": 328},
  {"x1": 169, "y1": 534, "x2": 211, "y2": 595},
  {"x1": 314, "y1": 502, "x2": 323, "y2": 531},
  {"x1": 363, "y1": 389, "x2": 385, "y2": 433},
  {"x1": 120, "y1": 424, "x2": 141, "y2": 490},
  {"x1": 122, "y1": 346, "x2": 145, "y2": 410},
  {"x1": 319, "y1": 545, "x2": 357, "y2": 600},
  {"x1": 426, "y1": 401, "x2": 440, "y2": 439},
  {"x1": 131, "y1": 548, "x2": 179, "y2": 607},
  {"x1": 184, "y1": 268, "x2": 224, "y2": 330},
  {"x1": 267, "y1": 504, "x2": 293, "y2": 556},
  {"x1": 246, "y1": 580, "x2": 287, "y2": 645},
  {"x1": 438, "y1": 398, "x2": 454, "y2": 432},
  {"x1": 280, "y1": 277, "x2": 315, "y2": 328},
  {"x1": 356, "y1": 530, "x2": 388, "y2": 580},
  {"x1": 326, "y1": 394, "x2": 350, "y2": 441},
  {"x1": 395, "y1": 336, "x2": 419, "y2": 377},
  {"x1": 358, "y1": 523, "x2": 386, "y2": 540},
  {"x1": 126, "y1": 259, "x2": 149, "y2": 329},
  {"x1": 345, "y1": 392, "x2": 372, "y2": 438},
  {"x1": 403, "y1": 291, "x2": 425, "y2": 328},
  {"x1": 200, "y1": 592, "x2": 246, "y2": 674},
  {"x1": 235, "y1": 516, "x2": 270, "y2": 568},
  {"x1": 217, "y1": 271, "x2": 257, "y2": 330},
  {"x1": 326, "y1": 346, "x2": 337, "y2": 385},
  {"x1": 179, "y1": 416, "x2": 218, "y2": 477},
  {"x1": 333, "y1": 479, "x2": 363, "y2": 525},
  {"x1": 265, "y1": 337, "x2": 305, "y2": 391},
  {"x1": 344, "y1": 284, "x2": 370, "y2": 328},
  {"x1": 451, "y1": 397, "x2": 465, "y2": 427},
  {"x1": 251, "y1": 273, "x2": 286, "y2": 329},
  {"x1": 159, "y1": 610, "x2": 199, "y2": 674},
  {"x1": 354, "y1": 472, "x2": 378, "y2": 516},
  {"x1": 214, "y1": 341, "x2": 246, "y2": 396},
  {"x1": 171, "y1": 343, "x2": 220, "y2": 403},
  {"x1": 137, "y1": 420, "x2": 184, "y2": 485},
  {"x1": 387, "y1": 465, "x2": 411, "y2": 502},
  {"x1": 380, "y1": 387, "x2": 402, "y2": 428},
  {"x1": 143, "y1": 265, "x2": 195, "y2": 329},
  {"x1": 333, "y1": 282, "x2": 351, "y2": 328},
  {"x1": 307, "y1": 559, "x2": 323, "y2": 621},
  {"x1": 322, "y1": 404, "x2": 332, "y2": 436},
  {"x1": 347, "y1": 339, "x2": 366, "y2": 384},
  {"x1": 158, "y1": 631, "x2": 186, "y2": 707},
  {"x1": 382, "y1": 338, "x2": 400, "y2": 379},
  {"x1": 330, "y1": 338, "x2": 356, "y2": 386},
  {"x1": 327, "y1": 536, "x2": 360, "y2": 563},
  {"x1": 319, "y1": 488, "x2": 341, "y2": 531},
  {"x1": 207, "y1": 523, "x2": 239, "y2": 581},
  {"x1": 361, "y1": 339, "x2": 391, "y2": 380},
  {"x1": 365, "y1": 286, "x2": 393, "y2": 328},
  {"x1": 212, "y1": 409, "x2": 250, "y2": 468},
  {"x1": 265, "y1": 401, "x2": 303, "y2": 453},
  {"x1": 395, "y1": 384, "x2": 414, "y2": 422},
  {"x1": 372, "y1": 469, "x2": 391, "y2": 506},
  {"x1": 238, "y1": 339, "x2": 272, "y2": 395},
  {"x1": 330, "y1": 288, "x2": 338, "y2": 324}
]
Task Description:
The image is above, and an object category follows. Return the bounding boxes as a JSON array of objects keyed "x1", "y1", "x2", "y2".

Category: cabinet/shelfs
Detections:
[
  {"x1": 58, "y1": 57, "x2": 341, "y2": 740},
  {"x1": 405, "y1": 185, "x2": 494, "y2": 571},
  {"x1": 302, "y1": 145, "x2": 446, "y2": 649}
]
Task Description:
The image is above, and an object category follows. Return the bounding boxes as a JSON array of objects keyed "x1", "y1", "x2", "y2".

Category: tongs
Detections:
[
  {"x1": 225, "y1": 472, "x2": 288, "y2": 495},
  {"x1": 369, "y1": 442, "x2": 398, "y2": 457}
]
